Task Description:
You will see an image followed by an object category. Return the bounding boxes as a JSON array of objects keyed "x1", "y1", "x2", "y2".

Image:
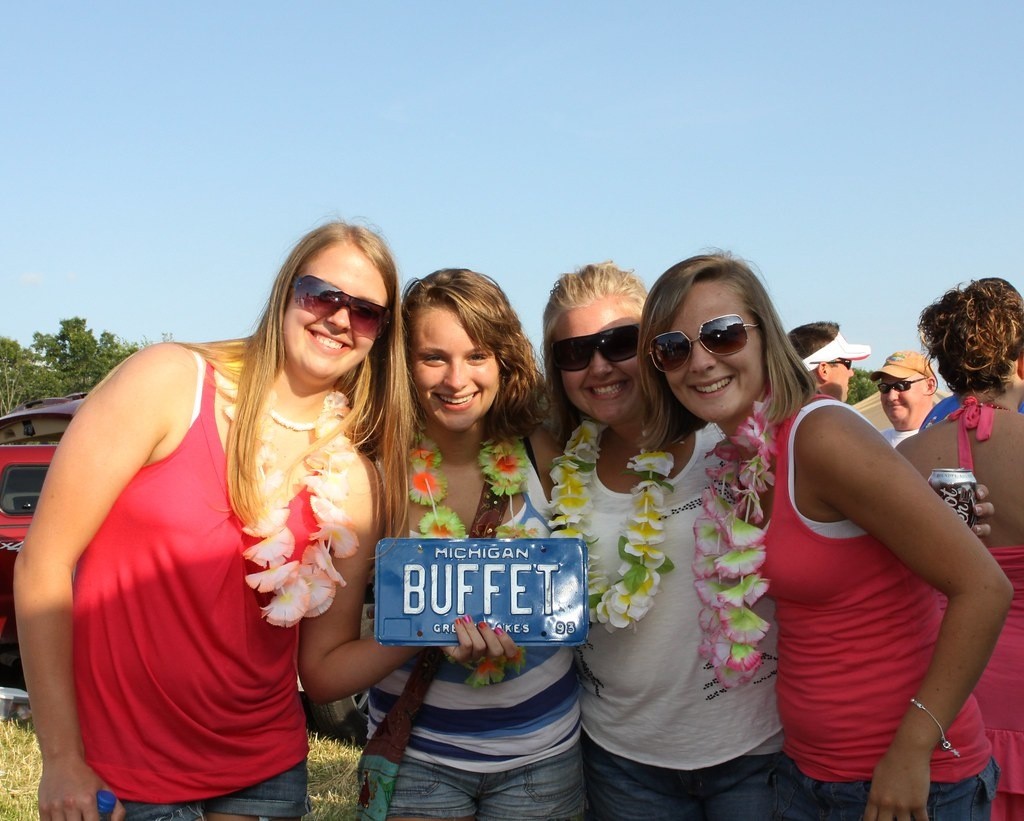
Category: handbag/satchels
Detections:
[{"x1": 355, "y1": 754, "x2": 401, "y2": 821}]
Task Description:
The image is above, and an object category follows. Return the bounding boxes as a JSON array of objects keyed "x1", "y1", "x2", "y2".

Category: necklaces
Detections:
[
  {"x1": 690, "y1": 389, "x2": 780, "y2": 687},
  {"x1": 547, "y1": 413, "x2": 675, "y2": 638},
  {"x1": 214, "y1": 368, "x2": 359, "y2": 624},
  {"x1": 408, "y1": 421, "x2": 532, "y2": 686}
]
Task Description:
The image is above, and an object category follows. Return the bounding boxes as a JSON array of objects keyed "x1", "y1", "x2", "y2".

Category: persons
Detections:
[
  {"x1": 542, "y1": 262, "x2": 784, "y2": 821},
  {"x1": 639, "y1": 250, "x2": 1024, "y2": 821},
  {"x1": 13, "y1": 221, "x2": 601, "y2": 821}
]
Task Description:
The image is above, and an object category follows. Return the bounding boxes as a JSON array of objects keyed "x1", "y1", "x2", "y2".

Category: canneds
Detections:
[{"x1": 927, "y1": 468, "x2": 978, "y2": 530}]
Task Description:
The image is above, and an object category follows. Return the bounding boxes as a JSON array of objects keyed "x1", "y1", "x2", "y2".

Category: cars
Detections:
[{"x1": 0, "y1": 390, "x2": 87, "y2": 648}]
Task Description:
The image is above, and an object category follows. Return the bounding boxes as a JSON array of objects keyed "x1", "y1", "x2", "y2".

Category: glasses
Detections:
[
  {"x1": 551, "y1": 324, "x2": 640, "y2": 371},
  {"x1": 878, "y1": 378, "x2": 926, "y2": 393},
  {"x1": 811, "y1": 359, "x2": 851, "y2": 370},
  {"x1": 649, "y1": 313, "x2": 759, "y2": 372},
  {"x1": 293, "y1": 275, "x2": 391, "y2": 339}
]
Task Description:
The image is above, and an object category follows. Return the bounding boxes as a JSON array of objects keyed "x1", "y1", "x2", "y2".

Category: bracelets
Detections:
[{"x1": 910, "y1": 699, "x2": 960, "y2": 758}]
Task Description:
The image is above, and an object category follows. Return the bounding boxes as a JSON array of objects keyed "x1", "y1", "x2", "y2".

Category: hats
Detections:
[
  {"x1": 802, "y1": 331, "x2": 871, "y2": 371},
  {"x1": 870, "y1": 350, "x2": 934, "y2": 381}
]
